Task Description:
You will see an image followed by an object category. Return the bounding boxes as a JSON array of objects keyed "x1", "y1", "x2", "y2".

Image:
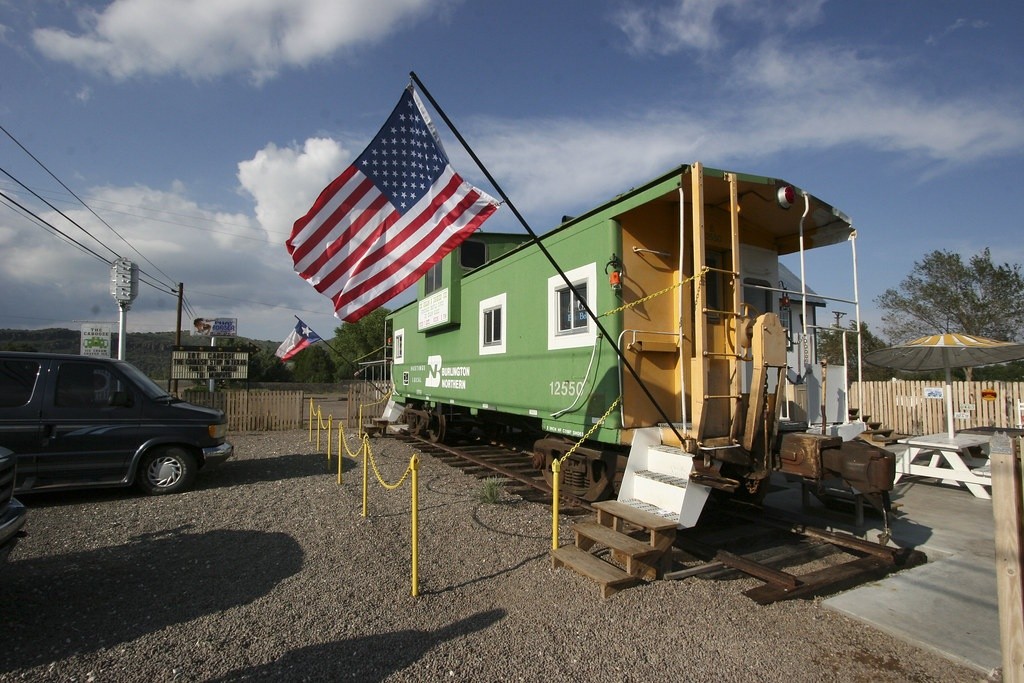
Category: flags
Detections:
[
  {"x1": 274, "y1": 320, "x2": 321, "y2": 363},
  {"x1": 286, "y1": 82, "x2": 504, "y2": 326}
]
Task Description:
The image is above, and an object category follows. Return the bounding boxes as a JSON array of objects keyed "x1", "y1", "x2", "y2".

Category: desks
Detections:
[{"x1": 891, "y1": 432, "x2": 993, "y2": 499}]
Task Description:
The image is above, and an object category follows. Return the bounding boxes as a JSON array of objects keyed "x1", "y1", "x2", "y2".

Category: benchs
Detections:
[{"x1": 971, "y1": 464, "x2": 992, "y2": 477}]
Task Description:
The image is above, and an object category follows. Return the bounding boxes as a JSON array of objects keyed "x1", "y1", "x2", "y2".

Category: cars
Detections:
[{"x1": 0, "y1": 446, "x2": 27, "y2": 544}]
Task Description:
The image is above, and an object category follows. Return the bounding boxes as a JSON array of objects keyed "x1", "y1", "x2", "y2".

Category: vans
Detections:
[{"x1": 1, "y1": 351, "x2": 233, "y2": 497}]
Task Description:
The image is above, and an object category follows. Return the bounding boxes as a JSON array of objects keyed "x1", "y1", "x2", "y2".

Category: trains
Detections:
[{"x1": 383, "y1": 164, "x2": 897, "y2": 550}]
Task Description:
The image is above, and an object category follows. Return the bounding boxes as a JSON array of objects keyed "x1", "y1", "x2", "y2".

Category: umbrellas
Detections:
[{"x1": 862, "y1": 333, "x2": 1024, "y2": 440}]
